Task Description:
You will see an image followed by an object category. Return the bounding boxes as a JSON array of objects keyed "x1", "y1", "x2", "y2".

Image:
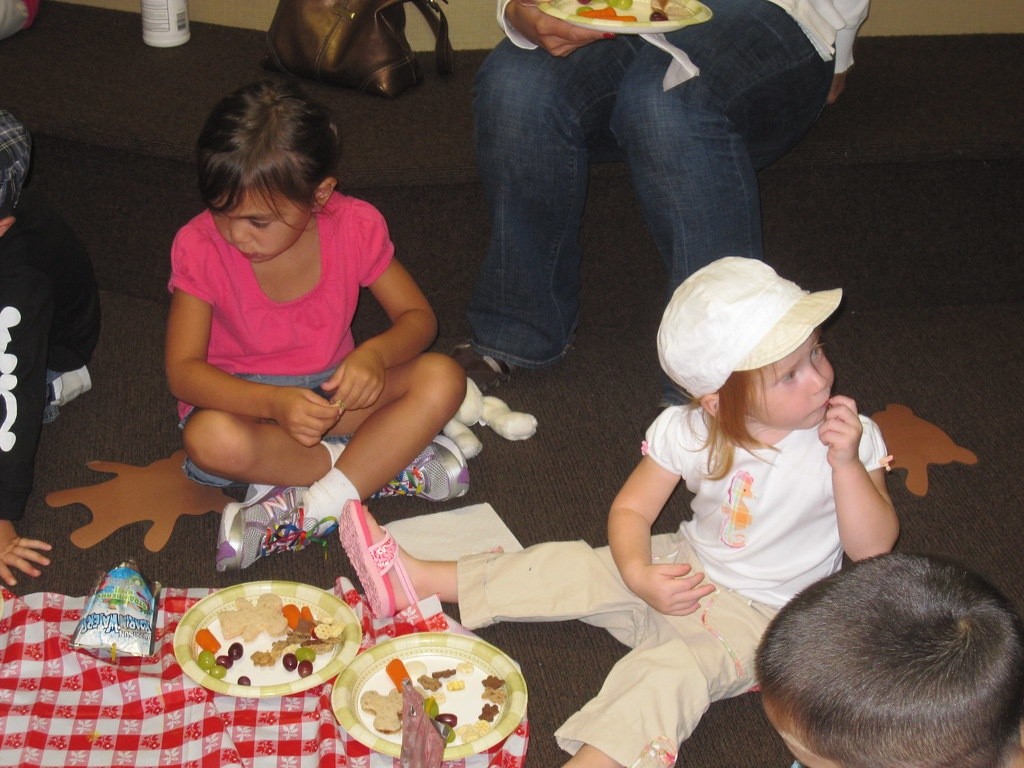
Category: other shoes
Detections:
[{"x1": 451, "y1": 346, "x2": 519, "y2": 393}]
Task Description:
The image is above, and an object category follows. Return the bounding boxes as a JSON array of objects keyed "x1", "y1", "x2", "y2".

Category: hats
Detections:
[
  {"x1": 0, "y1": 109, "x2": 31, "y2": 210},
  {"x1": 656, "y1": 256, "x2": 842, "y2": 400}
]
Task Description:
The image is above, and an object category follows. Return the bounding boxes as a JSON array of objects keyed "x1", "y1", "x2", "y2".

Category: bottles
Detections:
[{"x1": 141, "y1": 0, "x2": 191, "y2": 47}]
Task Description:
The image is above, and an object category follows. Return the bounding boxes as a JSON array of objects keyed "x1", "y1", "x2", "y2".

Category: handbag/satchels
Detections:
[{"x1": 260, "y1": 0, "x2": 453, "y2": 99}]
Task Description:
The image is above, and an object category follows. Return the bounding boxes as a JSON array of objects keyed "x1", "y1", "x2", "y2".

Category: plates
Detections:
[
  {"x1": 173, "y1": 579, "x2": 363, "y2": 699},
  {"x1": 331, "y1": 632, "x2": 528, "y2": 762},
  {"x1": 536, "y1": 0, "x2": 713, "y2": 34}
]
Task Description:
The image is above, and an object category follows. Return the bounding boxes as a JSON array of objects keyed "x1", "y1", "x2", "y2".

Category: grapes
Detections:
[
  {"x1": 575, "y1": 0, "x2": 633, "y2": 14},
  {"x1": 282, "y1": 647, "x2": 316, "y2": 678},
  {"x1": 197, "y1": 642, "x2": 250, "y2": 686},
  {"x1": 423, "y1": 697, "x2": 457, "y2": 743},
  {"x1": 648, "y1": 12, "x2": 668, "y2": 21}
]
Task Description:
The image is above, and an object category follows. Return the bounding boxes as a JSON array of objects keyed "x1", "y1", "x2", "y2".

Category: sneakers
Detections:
[
  {"x1": 340, "y1": 434, "x2": 469, "y2": 503},
  {"x1": 215, "y1": 483, "x2": 338, "y2": 571}
]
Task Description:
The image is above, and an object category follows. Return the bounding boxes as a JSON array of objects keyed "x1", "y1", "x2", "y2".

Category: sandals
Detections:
[{"x1": 339, "y1": 500, "x2": 421, "y2": 618}]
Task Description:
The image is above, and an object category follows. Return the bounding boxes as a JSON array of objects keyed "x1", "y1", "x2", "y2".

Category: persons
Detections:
[
  {"x1": 754, "y1": 552, "x2": 1024, "y2": 768},
  {"x1": 340, "y1": 254, "x2": 898, "y2": 768},
  {"x1": 164, "y1": 79, "x2": 470, "y2": 572},
  {"x1": 1, "y1": 112, "x2": 101, "y2": 585},
  {"x1": 434, "y1": 0, "x2": 871, "y2": 434}
]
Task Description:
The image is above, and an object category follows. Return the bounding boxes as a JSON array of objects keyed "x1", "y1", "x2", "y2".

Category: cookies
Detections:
[
  {"x1": 362, "y1": 661, "x2": 507, "y2": 744},
  {"x1": 216, "y1": 593, "x2": 289, "y2": 643},
  {"x1": 251, "y1": 611, "x2": 345, "y2": 668}
]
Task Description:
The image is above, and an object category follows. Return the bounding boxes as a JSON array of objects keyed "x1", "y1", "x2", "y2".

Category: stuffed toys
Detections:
[{"x1": 444, "y1": 377, "x2": 539, "y2": 458}]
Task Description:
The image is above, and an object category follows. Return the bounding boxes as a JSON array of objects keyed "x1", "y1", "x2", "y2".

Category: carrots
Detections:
[
  {"x1": 282, "y1": 604, "x2": 317, "y2": 637},
  {"x1": 386, "y1": 658, "x2": 412, "y2": 692},
  {"x1": 196, "y1": 629, "x2": 221, "y2": 655},
  {"x1": 577, "y1": 7, "x2": 637, "y2": 21}
]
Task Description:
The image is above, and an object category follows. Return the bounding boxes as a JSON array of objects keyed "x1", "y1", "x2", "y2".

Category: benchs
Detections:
[{"x1": 0, "y1": 0, "x2": 1024, "y2": 339}]
